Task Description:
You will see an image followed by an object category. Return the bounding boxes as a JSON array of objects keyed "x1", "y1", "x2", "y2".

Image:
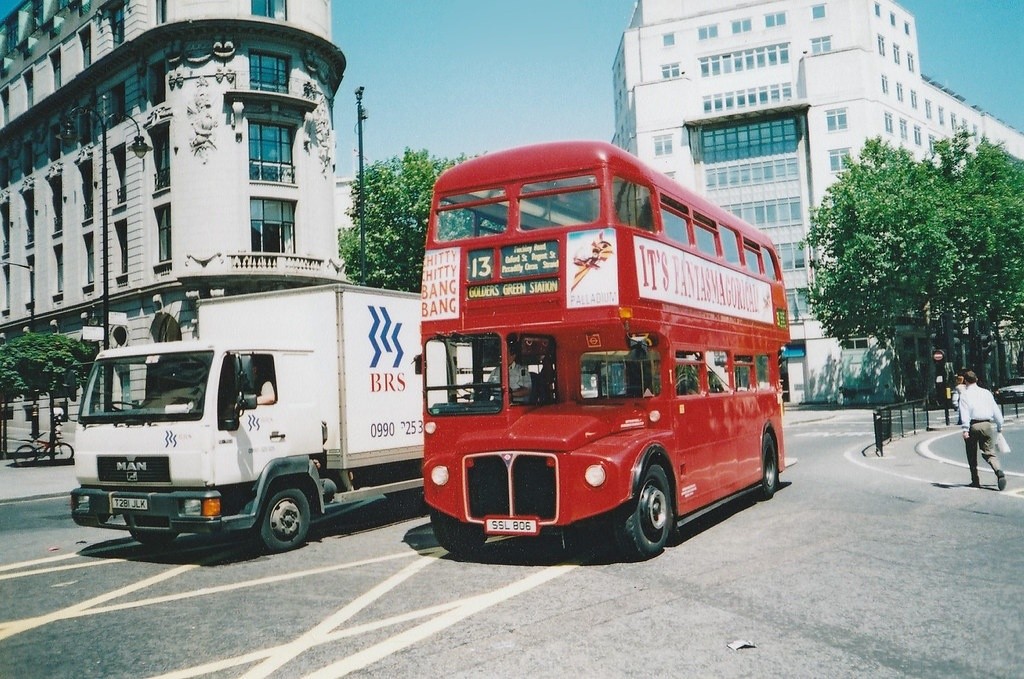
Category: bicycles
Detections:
[{"x1": 13, "y1": 413, "x2": 75, "y2": 466}]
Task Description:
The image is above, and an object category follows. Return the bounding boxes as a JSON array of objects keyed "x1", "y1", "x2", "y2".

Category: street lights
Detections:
[
  {"x1": 52, "y1": 96, "x2": 154, "y2": 417},
  {"x1": 354, "y1": 86, "x2": 370, "y2": 287}
]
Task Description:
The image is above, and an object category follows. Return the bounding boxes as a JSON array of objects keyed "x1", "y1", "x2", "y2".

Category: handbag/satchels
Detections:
[{"x1": 995, "y1": 432, "x2": 1011, "y2": 453}]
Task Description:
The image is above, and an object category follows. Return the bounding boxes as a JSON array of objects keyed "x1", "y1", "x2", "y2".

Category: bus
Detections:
[{"x1": 416, "y1": 139, "x2": 797, "y2": 556}]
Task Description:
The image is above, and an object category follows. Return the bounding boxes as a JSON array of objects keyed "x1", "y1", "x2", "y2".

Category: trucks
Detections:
[{"x1": 67, "y1": 280, "x2": 555, "y2": 548}]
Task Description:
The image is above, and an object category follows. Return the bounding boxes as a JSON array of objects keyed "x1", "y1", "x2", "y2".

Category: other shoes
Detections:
[
  {"x1": 969, "y1": 482, "x2": 979, "y2": 487},
  {"x1": 997, "y1": 470, "x2": 1006, "y2": 491}
]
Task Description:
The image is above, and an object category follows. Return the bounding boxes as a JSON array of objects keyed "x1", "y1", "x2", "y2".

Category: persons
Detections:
[
  {"x1": 476, "y1": 341, "x2": 532, "y2": 403},
  {"x1": 957, "y1": 371, "x2": 1007, "y2": 491},
  {"x1": 235, "y1": 356, "x2": 274, "y2": 405},
  {"x1": 54, "y1": 402, "x2": 64, "y2": 439}
]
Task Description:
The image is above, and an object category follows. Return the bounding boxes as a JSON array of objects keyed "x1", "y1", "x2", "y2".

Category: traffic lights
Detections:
[
  {"x1": 978, "y1": 335, "x2": 991, "y2": 353},
  {"x1": 944, "y1": 313, "x2": 960, "y2": 353},
  {"x1": 931, "y1": 349, "x2": 946, "y2": 368}
]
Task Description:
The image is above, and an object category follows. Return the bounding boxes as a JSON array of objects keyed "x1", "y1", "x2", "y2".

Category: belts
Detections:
[{"x1": 971, "y1": 420, "x2": 989, "y2": 423}]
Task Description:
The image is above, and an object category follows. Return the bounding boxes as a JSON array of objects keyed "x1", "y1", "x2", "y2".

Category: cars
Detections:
[{"x1": 997, "y1": 377, "x2": 1024, "y2": 402}]
